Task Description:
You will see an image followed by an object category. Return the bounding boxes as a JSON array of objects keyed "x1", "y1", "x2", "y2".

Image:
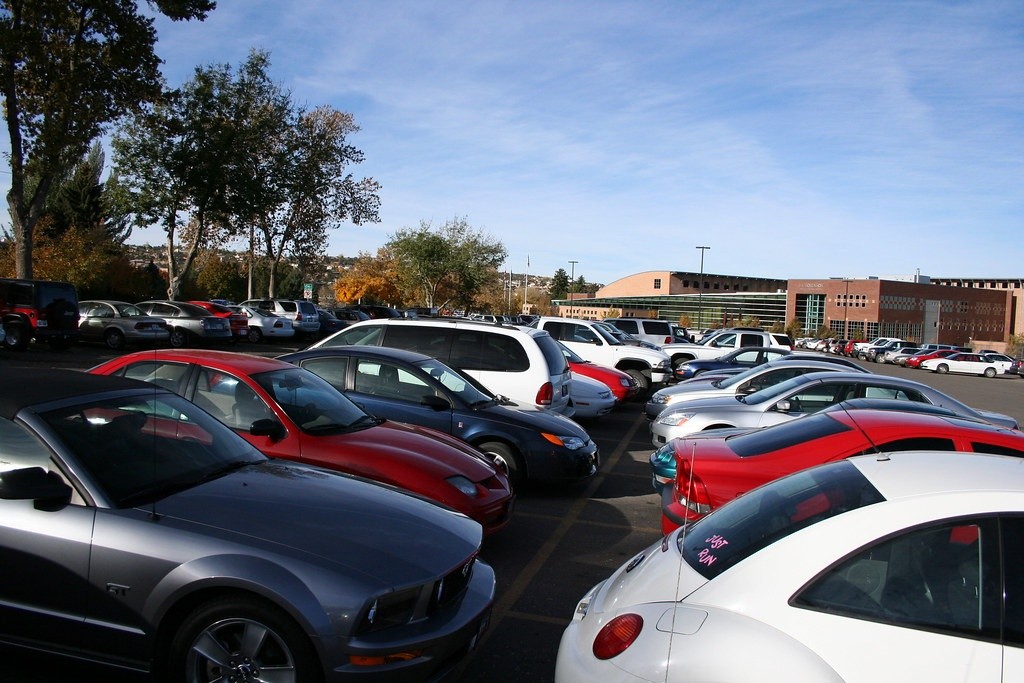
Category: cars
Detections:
[
  {"x1": 1, "y1": 371, "x2": 501, "y2": 683},
  {"x1": 77, "y1": 286, "x2": 1024, "y2": 543},
  {"x1": 555, "y1": 453, "x2": 1023, "y2": 683}
]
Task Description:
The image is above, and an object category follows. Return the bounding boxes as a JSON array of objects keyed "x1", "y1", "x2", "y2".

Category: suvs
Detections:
[{"x1": 0, "y1": 277, "x2": 80, "y2": 352}]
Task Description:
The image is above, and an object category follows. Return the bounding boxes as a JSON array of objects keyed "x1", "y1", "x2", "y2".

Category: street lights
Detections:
[
  {"x1": 568, "y1": 260, "x2": 578, "y2": 318},
  {"x1": 841, "y1": 280, "x2": 854, "y2": 340},
  {"x1": 695, "y1": 246, "x2": 711, "y2": 329}
]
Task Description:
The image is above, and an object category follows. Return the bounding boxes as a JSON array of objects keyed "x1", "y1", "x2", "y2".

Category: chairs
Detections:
[{"x1": 230, "y1": 382, "x2": 268, "y2": 422}]
